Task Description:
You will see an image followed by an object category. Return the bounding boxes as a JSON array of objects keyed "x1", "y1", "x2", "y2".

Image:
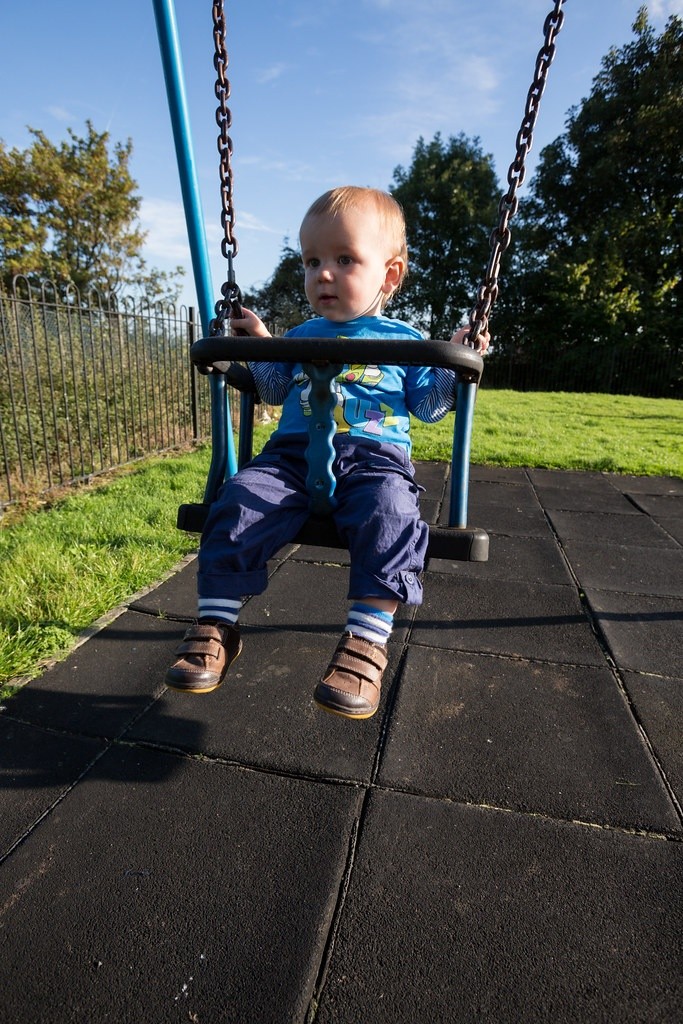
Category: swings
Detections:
[{"x1": 173, "y1": 0, "x2": 572, "y2": 564}]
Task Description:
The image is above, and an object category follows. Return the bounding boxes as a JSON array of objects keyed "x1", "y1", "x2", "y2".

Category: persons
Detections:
[{"x1": 164, "y1": 185, "x2": 490, "y2": 715}]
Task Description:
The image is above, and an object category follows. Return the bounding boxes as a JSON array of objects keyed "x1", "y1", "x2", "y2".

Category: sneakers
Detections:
[
  {"x1": 313, "y1": 630, "x2": 389, "y2": 720},
  {"x1": 164, "y1": 618, "x2": 243, "y2": 694}
]
